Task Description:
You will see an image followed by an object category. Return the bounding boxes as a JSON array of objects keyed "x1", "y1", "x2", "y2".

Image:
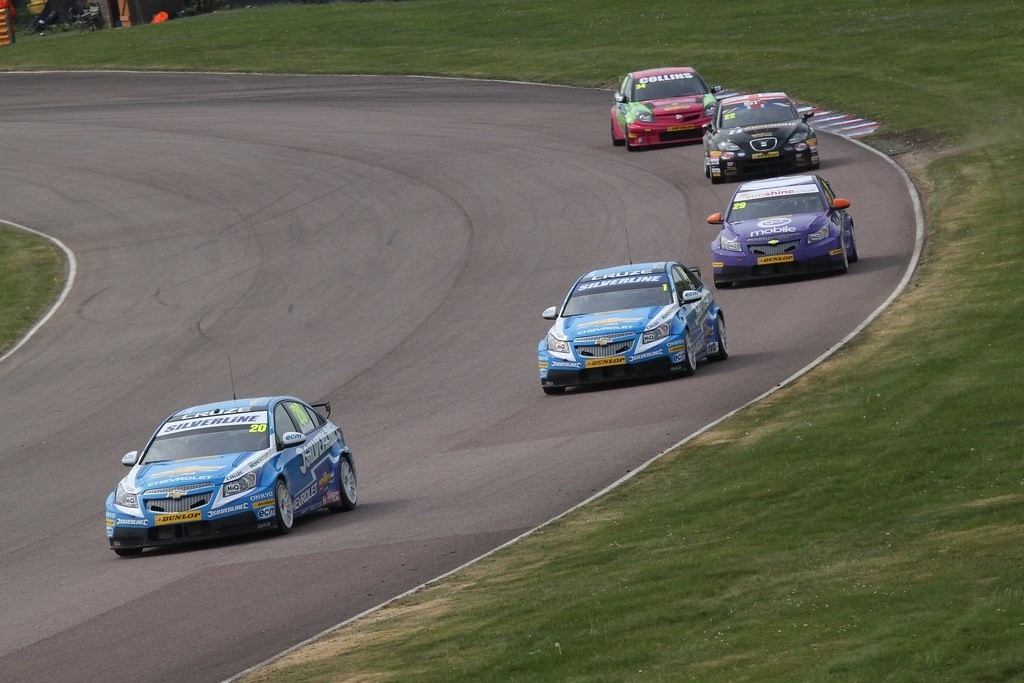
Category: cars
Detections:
[
  {"x1": 106, "y1": 396, "x2": 357, "y2": 556},
  {"x1": 702, "y1": 92, "x2": 820, "y2": 184},
  {"x1": 706, "y1": 174, "x2": 857, "y2": 288},
  {"x1": 538, "y1": 259, "x2": 728, "y2": 395},
  {"x1": 610, "y1": 67, "x2": 722, "y2": 151}
]
